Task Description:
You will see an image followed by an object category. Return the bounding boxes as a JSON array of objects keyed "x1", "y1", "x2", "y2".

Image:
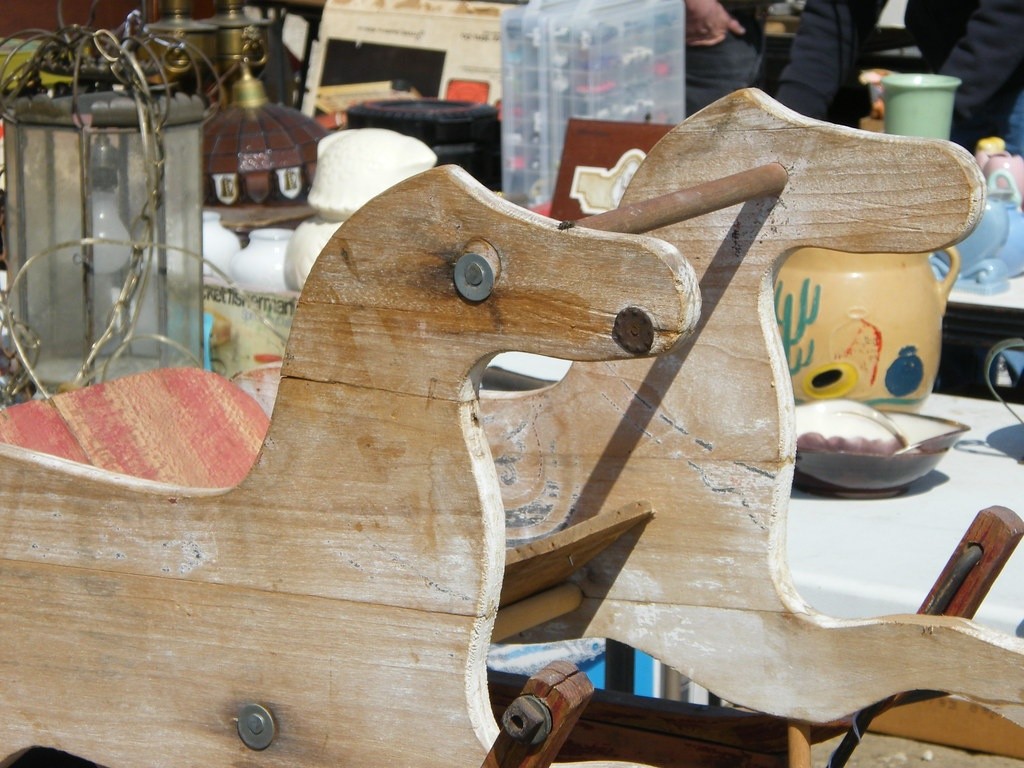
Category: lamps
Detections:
[{"x1": 3, "y1": 7, "x2": 205, "y2": 395}]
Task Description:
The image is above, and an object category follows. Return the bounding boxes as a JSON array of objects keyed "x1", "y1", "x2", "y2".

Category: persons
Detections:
[{"x1": 685, "y1": 0, "x2": 1024, "y2": 168}]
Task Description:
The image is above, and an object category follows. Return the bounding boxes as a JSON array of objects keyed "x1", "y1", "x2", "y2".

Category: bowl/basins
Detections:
[{"x1": 794, "y1": 397, "x2": 971, "y2": 498}]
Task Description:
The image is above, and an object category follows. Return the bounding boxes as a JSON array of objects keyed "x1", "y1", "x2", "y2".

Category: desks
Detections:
[{"x1": 654, "y1": 391, "x2": 1024, "y2": 699}]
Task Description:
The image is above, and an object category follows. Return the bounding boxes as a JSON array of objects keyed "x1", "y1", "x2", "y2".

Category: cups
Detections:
[
  {"x1": 880, "y1": 74, "x2": 962, "y2": 141},
  {"x1": 984, "y1": 339, "x2": 1024, "y2": 462}
]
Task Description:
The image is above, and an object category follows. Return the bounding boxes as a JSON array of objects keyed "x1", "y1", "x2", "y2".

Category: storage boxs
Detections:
[{"x1": 500, "y1": 0, "x2": 687, "y2": 210}]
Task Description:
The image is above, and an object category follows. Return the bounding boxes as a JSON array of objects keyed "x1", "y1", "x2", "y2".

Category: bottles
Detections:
[
  {"x1": 228, "y1": 228, "x2": 293, "y2": 292},
  {"x1": 200, "y1": 210, "x2": 241, "y2": 286},
  {"x1": 131, "y1": 224, "x2": 183, "y2": 358}
]
